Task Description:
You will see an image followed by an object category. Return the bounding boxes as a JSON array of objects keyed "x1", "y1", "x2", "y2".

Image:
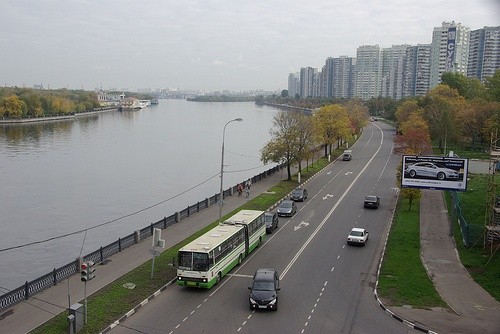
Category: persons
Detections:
[
  {"x1": 237, "y1": 183, "x2": 243, "y2": 197},
  {"x1": 245, "y1": 178, "x2": 252, "y2": 198}
]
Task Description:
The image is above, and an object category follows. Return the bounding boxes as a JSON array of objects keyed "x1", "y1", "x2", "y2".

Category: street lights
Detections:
[{"x1": 219, "y1": 117, "x2": 244, "y2": 220}]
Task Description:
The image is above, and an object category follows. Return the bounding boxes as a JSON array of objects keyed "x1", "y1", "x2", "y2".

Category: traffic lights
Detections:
[
  {"x1": 88, "y1": 261, "x2": 96, "y2": 280},
  {"x1": 80, "y1": 262, "x2": 89, "y2": 282}
]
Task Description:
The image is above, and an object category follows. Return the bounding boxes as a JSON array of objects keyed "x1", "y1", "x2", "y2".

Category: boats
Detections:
[{"x1": 118, "y1": 98, "x2": 158, "y2": 111}]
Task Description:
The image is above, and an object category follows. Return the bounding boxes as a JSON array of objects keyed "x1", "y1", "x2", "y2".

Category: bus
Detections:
[{"x1": 173, "y1": 210, "x2": 266, "y2": 289}]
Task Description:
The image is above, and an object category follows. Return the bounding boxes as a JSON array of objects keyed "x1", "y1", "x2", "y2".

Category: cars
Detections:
[
  {"x1": 342, "y1": 153, "x2": 352, "y2": 161},
  {"x1": 370, "y1": 118, "x2": 379, "y2": 122},
  {"x1": 265, "y1": 212, "x2": 279, "y2": 234},
  {"x1": 406, "y1": 161, "x2": 460, "y2": 180},
  {"x1": 347, "y1": 228, "x2": 369, "y2": 246},
  {"x1": 364, "y1": 195, "x2": 380, "y2": 209},
  {"x1": 290, "y1": 188, "x2": 308, "y2": 202},
  {"x1": 277, "y1": 200, "x2": 297, "y2": 217}
]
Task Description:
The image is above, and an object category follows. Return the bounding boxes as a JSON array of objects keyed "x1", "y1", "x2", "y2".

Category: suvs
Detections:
[{"x1": 247, "y1": 267, "x2": 281, "y2": 310}]
